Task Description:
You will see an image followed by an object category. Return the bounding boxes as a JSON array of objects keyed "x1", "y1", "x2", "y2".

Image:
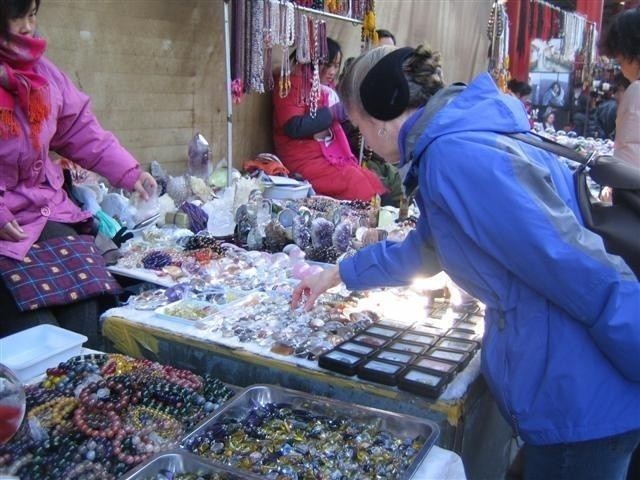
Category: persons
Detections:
[
  {"x1": 538, "y1": 106, "x2": 563, "y2": 134},
  {"x1": 0, "y1": 0, "x2": 159, "y2": 353},
  {"x1": 592, "y1": 79, "x2": 628, "y2": 142},
  {"x1": 273, "y1": 35, "x2": 396, "y2": 211},
  {"x1": 542, "y1": 81, "x2": 566, "y2": 108},
  {"x1": 596, "y1": 6, "x2": 640, "y2": 175},
  {"x1": 290, "y1": 42, "x2": 640, "y2": 480},
  {"x1": 506, "y1": 77, "x2": 533, "y2": 106}
]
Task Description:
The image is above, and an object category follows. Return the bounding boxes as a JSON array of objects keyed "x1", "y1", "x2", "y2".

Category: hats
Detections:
[{"x1": 543, "y1": 106, "x2": 557, "y2": 119}]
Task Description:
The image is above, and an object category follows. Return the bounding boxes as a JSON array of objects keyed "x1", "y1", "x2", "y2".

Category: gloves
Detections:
[{"x1": 331, "y1": 100, "x2": 348, "y2": 121}]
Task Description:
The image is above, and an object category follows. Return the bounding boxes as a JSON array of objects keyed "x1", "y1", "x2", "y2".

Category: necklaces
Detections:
[
  {"x1": 229, "y1": 1, "x2": 379, "y2": 123},
  {"x1": 486, "y1": 0, "x2": 597, "y2": 100}
]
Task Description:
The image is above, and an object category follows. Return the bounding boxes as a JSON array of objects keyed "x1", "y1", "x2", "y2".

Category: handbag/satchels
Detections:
[{"x1": 574, "y1": 152, "x2": 640, "y2": 278}]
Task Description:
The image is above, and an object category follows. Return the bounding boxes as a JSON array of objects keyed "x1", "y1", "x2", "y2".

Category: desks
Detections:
[{"x1": 0, "y1": 247, "x2": 506, "y2": 480}]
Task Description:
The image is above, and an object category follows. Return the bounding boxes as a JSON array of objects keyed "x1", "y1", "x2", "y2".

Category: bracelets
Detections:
[
  {"x1": 121, "y1": 225, "x2": 225, "y2": 271},
  {"x1": 0, "y1": 352, "x2": 237, "y2": 480}
]
